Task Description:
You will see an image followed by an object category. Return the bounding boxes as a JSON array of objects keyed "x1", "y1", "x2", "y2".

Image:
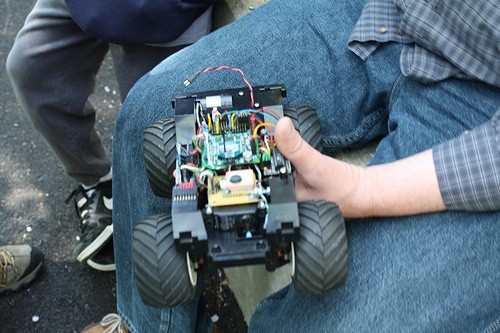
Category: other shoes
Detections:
[{"x1": 82, "y1": 312, "x2": 132, "y2": 333}]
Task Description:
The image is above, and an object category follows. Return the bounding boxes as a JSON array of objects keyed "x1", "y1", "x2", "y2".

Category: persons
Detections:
[
  {"x1": 5, "y1": 0, "x2": 213, "y2": 272},
  {"x1": 80, "y1": 0, "x2": 500, "y2": 333},
  {"x1": 0, "y1": 244, "x2": 46, "y2": 294}
]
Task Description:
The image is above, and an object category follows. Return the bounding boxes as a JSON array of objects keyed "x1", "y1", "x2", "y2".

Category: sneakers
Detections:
[
  {"x1": 0, "y1": 243, "x2": 45, "y2": 292},
  {"x1": 64, "y1": 177, "x2": 113, "y2": 263},
  {"x1": 87, "y1": 238, "x2": 116, "y2": 272}
]
